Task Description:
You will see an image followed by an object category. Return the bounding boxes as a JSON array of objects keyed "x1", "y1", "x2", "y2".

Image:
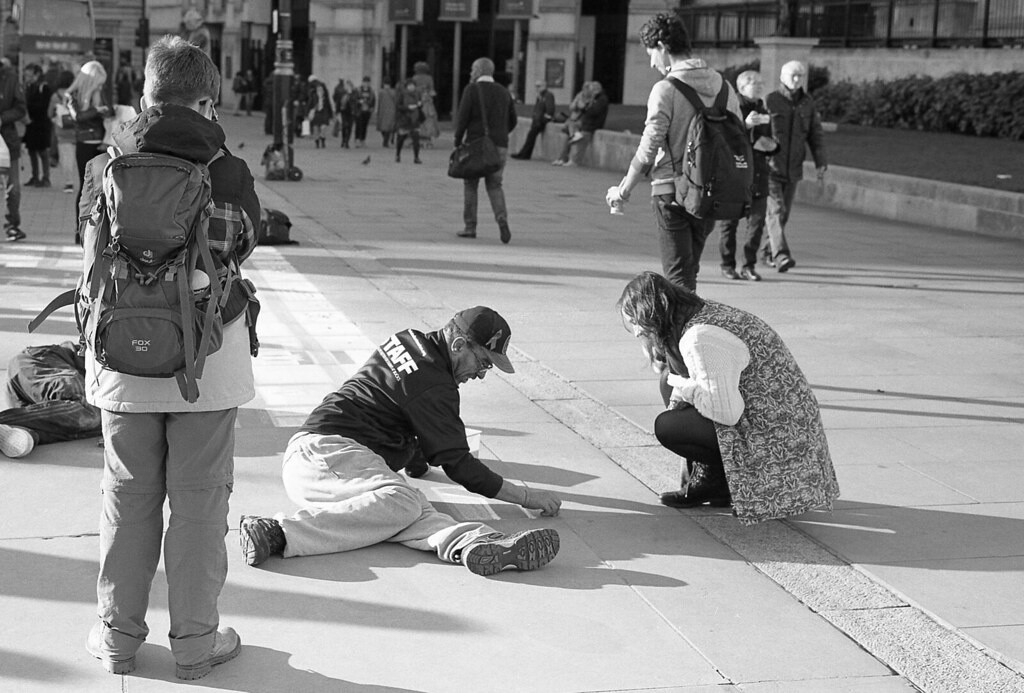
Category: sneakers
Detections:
[
  {"x1": 462, "y1": 527, "x2": 560, "y2": 576},
  {"x1": 238, "y1": 513, "x2": 284, "y2": 567}
]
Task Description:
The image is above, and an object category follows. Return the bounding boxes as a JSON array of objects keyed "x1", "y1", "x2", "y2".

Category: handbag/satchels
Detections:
[
  {"x1": 102, "y1": 104, "x2": 138, "y2": 147},
  {"x1": 301, "y1": 119, "x2": 311, "y2": 136},
  {"x1": 419, "y1": 108, "x2": 426, "y2": 124},
  {"x1": 447, "y1": 135, "x2": 502, "y2": 179}
]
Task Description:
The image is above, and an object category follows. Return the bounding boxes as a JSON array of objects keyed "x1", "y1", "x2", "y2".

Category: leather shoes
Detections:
[
  {"x1": 176, "y1": 626, "x2": 241, "y2": 680},
  {"x1": 86, "y1": 623, "x2": 137, "y2": 675}
]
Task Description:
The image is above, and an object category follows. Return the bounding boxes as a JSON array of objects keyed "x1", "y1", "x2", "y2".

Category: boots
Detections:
[
  {"x1": 658, "y1": 463, "x2": 732, "y2": 507},
  {"x1": 314, "y1": 136, "x2": 326, "y2": 148}
]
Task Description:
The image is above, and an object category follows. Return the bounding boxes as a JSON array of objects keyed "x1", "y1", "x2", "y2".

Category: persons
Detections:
[
  {"x1": 511, "y1": 80, "x2": 555, "y2": 160},
  {"x1": 617, "y1": 272, "x2": 840, "y2": 527},
  {"x1": 239, "y1": 306, "x2": 562, "y2": 576},
  {"x1": 719, "y1": 69, "x2": 780, "y2": 281},
  {"x1": 604, "y1": 14, "x2": 742, "y2": 374},
  {"x1": 0, "y1": 341, "x2": 101, "y2": 459},
  {"x1": 70, "y1": 35, "x2": 262, "y2": 679},
  {"x1": 453, "y1": 57, "x2": 517, "y2": 244},
  {"x1": 231, "y1": 61, "x2": 441, "y2": 165},
  {"x1": 564, "y1": 82, "x2": 608, "y2": 168},
  {"x1": 0, "y1": 55, "x2": 147, "y2": 246},
  {"x1": 550, "y1": 81, "x2": 590, "y2": 166},
  {"x1": 762, "y1": 60, "x2": 829, "y2": 274}
]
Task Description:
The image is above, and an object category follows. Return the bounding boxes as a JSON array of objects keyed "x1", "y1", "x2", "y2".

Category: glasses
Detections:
[
  {"x1": 199, "y1": 99, "x2": 220, "y2": 123},
  {"x1": 470, "y1": 348, "x2": 494, "y2": 371}
]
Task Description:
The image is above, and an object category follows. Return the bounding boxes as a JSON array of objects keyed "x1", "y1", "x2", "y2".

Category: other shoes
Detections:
[
  {"x1": 551, "y1": 159, "x2": 578, "y2": 168},
  {"x1": 62, "y1": 185, "x2": 74, "y2": 193},
  {"x1": 498, "y1": 216, "x2": 511, "y2": 244},
  {"x1": 762, "y1": 252, "x2": 795, "y2": 272},
  {"x1": 24, "y1": 178, "x2": 51, "y2": 189},
  {"x1": 568, "y1": 134, "x2": 584, "y2": 144},
  {"x1": 723, "y1": 265, "x2": 761, "y2": 282},
  {"x1": 0, "y1": 424, "x2": 38, "y2": 458},
  {"x1": 456, "y1": 230, "x2": 476, "y2": 239},
  {"x1": 4, "y1": 227, "x2": 25, "y2": 242}
]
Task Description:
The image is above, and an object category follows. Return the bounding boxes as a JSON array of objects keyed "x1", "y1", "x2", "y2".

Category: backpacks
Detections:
[
  {"x1": 663, "y1": 72, "x2": 756, "y2": 221},
  {"x1": 259, "y1": 207, "x2": 299, "y2": 244},
  {"x1": 51, "y1": 90, "x2": 76, "y2": 129},
  {"x1": 74, "y1": 146, "x2": 261, "y2": 404}
]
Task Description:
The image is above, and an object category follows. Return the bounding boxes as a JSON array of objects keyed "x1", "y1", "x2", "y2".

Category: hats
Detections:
[
  {"x1": 308, "y1": 74, "x2": 317, "y2": 82},
  {"x1": 452, "y1": 306, "x2": 515, "y2": 373}
]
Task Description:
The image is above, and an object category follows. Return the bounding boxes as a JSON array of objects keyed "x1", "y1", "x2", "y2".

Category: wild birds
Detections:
[
  {"x1": 238, "y1": 142, "x2": 245, "y2": 148},
  {"x1": 362, "y1": 154, "x2": 371, "y2": 165}
]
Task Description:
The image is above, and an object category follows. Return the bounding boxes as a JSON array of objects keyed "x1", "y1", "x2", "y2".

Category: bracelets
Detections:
[{"x1": 522, "y1": 486, "x2": 530, "y2": 508}]
